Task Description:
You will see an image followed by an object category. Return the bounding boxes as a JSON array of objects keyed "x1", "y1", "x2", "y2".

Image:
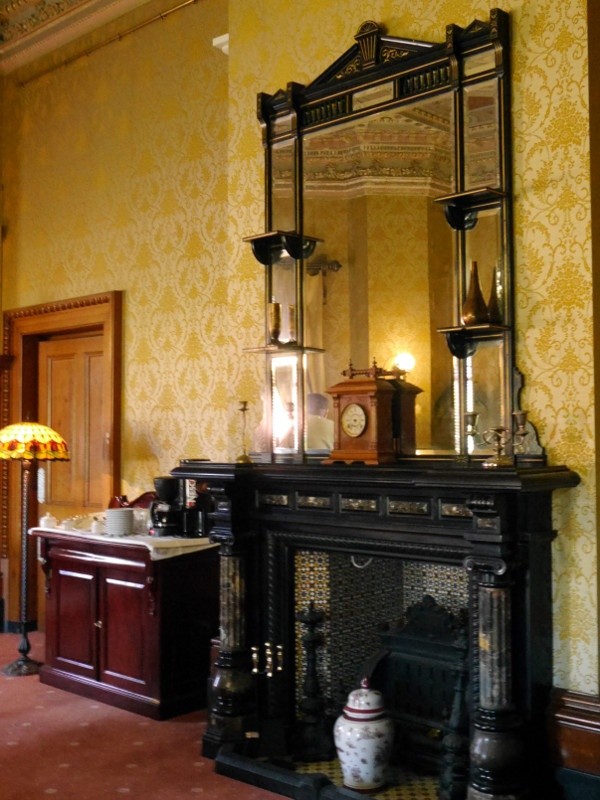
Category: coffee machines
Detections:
[{"x1": 149, "y1": 476, "x2": 178, "y2": 537}]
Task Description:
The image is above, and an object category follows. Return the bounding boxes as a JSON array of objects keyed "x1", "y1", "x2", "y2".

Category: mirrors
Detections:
[{"x1": 257, "y1": 7, "x2": 546, "y2": 455}]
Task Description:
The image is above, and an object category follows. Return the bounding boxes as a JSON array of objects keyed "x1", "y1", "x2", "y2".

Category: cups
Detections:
[{"x1": 105, "y1": 509, "x2": 134, "y2": 534}]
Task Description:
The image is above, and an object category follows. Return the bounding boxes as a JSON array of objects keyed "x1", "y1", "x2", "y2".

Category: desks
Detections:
[{"x1": 171, "y1": 459, "x2": 580, "y2": 800}]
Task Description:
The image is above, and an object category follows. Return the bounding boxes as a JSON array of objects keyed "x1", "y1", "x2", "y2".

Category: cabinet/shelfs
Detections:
[
  {"x1": 28, "y1": 527, "x2": 219, "y2": 721},
  {"x1": 243, "y1": 230, "x2": 300, "y2": 354},
  {"x1": 434, "y1": 186, "x2": 507, "y2": 357}
]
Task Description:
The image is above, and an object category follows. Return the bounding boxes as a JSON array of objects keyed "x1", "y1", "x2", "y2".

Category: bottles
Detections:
[
  {"x1": 462, "y1": 261, "x2": 488, "y2": 325},
  {"x1": 334, "y1": 677, "x2": 395, "y2": 792}
]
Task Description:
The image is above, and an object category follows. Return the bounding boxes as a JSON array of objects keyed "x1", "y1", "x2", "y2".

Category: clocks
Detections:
[
  {"x1": 387, "y1": 365, "x2": 424, "y2": 455},
  {"x1": 325, "y1": 356, "x2": 397, "y2": 465}
]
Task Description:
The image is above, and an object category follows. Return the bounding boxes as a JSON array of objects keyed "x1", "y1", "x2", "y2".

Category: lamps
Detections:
[{"x1": 0, "y1": 421, "x2": 72, "y2": 677}]
